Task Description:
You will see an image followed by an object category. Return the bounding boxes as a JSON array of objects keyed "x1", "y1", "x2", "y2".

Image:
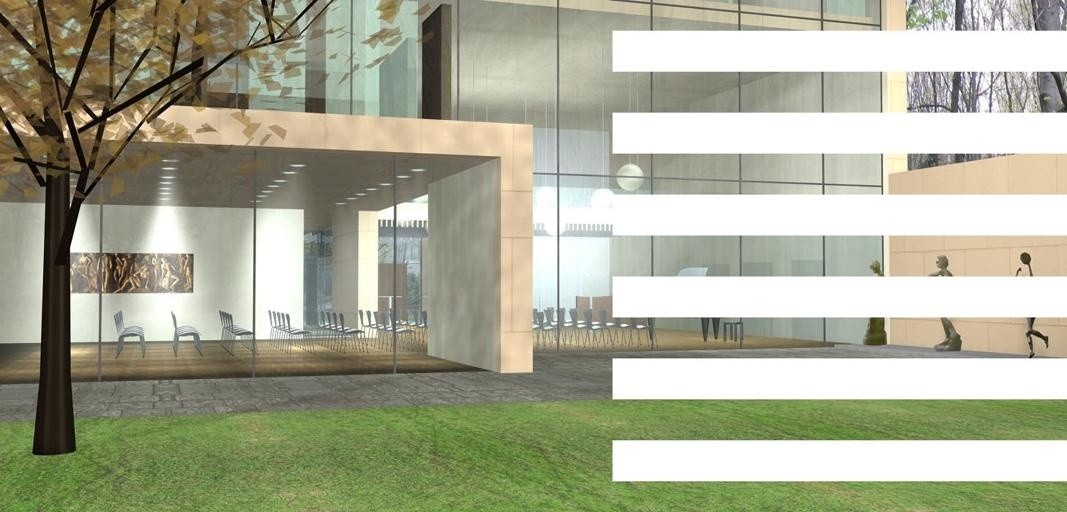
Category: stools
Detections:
[{"x1": 724, "y1": 321, "x2": 743, "y2": 342}]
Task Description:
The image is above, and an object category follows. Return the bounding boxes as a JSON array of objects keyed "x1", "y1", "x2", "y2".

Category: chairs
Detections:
[{"x1": 114, "y1": 308, "x2": 660, "y2": 357}]
